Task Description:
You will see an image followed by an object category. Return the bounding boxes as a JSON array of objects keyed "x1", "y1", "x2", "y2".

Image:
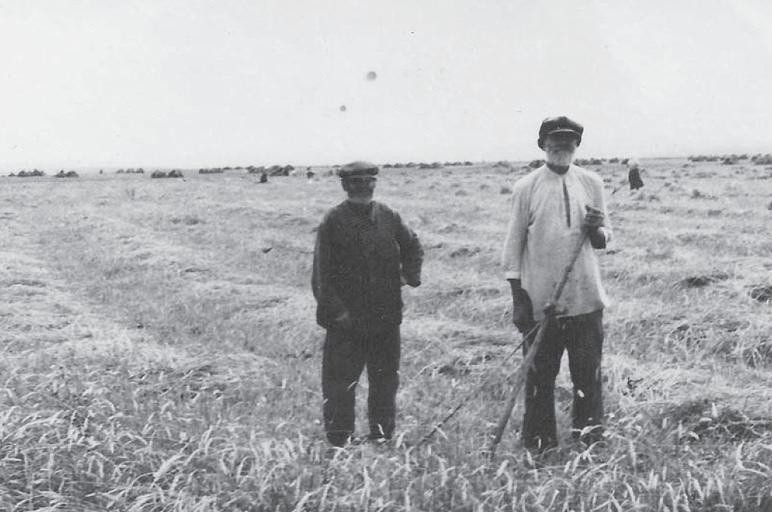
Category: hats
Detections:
[
  {"x1": 538, "y1": 115, "x2": 584, "y2": 139},
  {"x1": 339, "y1": 161, "x2": 380, "y2": 181}
]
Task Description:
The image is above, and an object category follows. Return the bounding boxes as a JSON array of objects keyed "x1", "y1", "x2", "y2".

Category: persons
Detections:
[
  {"x1": 496, "y1": 115, "x2": 614, "y2": 471},
  {"x1": 311, "y1": 157, "x2": 424, "y2": 448}
]
